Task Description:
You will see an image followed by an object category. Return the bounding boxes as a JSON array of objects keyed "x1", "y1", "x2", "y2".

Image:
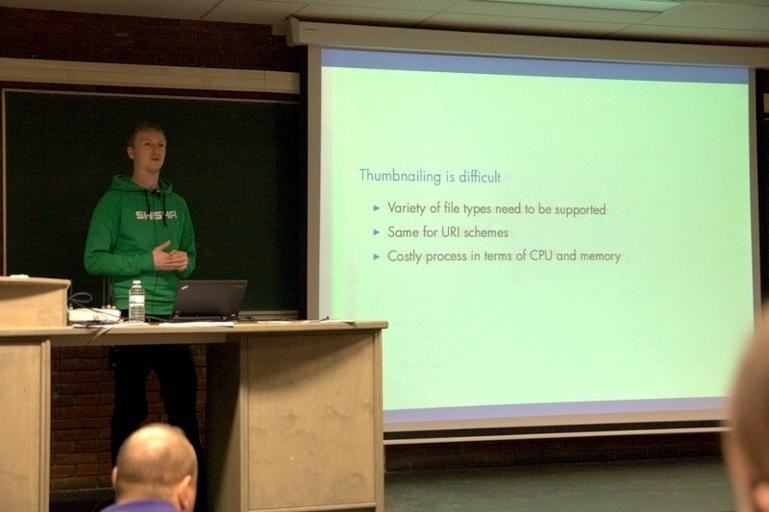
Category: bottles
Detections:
[{"x1": 128, "y1": 280, "x2": 145, "y2": 327}]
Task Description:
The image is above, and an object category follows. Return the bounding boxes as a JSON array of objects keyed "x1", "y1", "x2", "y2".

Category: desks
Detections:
[{"x1": 1, "y1": 318, "x2": 390, "y2": 511}]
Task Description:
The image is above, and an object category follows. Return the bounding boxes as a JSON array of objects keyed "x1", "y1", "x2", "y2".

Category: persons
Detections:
[
  {"x1": 718, "y1": 299, "x2": 769, "y2": 512},
  {"x1": 83, "y1": 120, "x2": 202, "y2": 465},
  {"x1": 98, "y1": 422, "x2": 199, "y2": 512}
]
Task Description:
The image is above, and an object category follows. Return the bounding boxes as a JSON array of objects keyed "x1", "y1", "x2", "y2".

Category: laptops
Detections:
[{"x1": 169, "y1": 280, "x2": 248, "y2": 322}]
